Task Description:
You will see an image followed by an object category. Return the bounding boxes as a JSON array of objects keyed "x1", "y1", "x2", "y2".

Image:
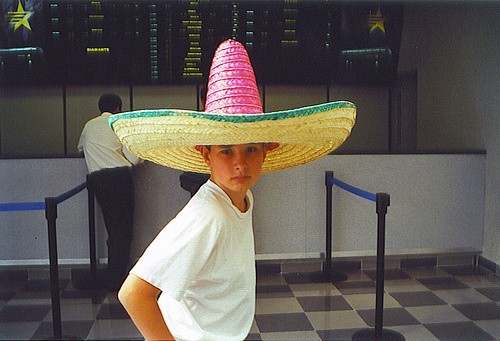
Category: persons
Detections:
[
  {"x1": 187, "y1": 83, "x2": 210, "y2": 198},
  {"x1": 105, "y1": 39, "x2": 357, "y2": 341},
  {"x1": 76, "y1": 92, "x2": 145, "y2": 293}
]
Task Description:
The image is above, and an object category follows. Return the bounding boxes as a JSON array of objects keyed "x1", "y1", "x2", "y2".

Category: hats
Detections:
[{"x1": 109, "y1": 38, "x2": 356, "y2": 174}]
